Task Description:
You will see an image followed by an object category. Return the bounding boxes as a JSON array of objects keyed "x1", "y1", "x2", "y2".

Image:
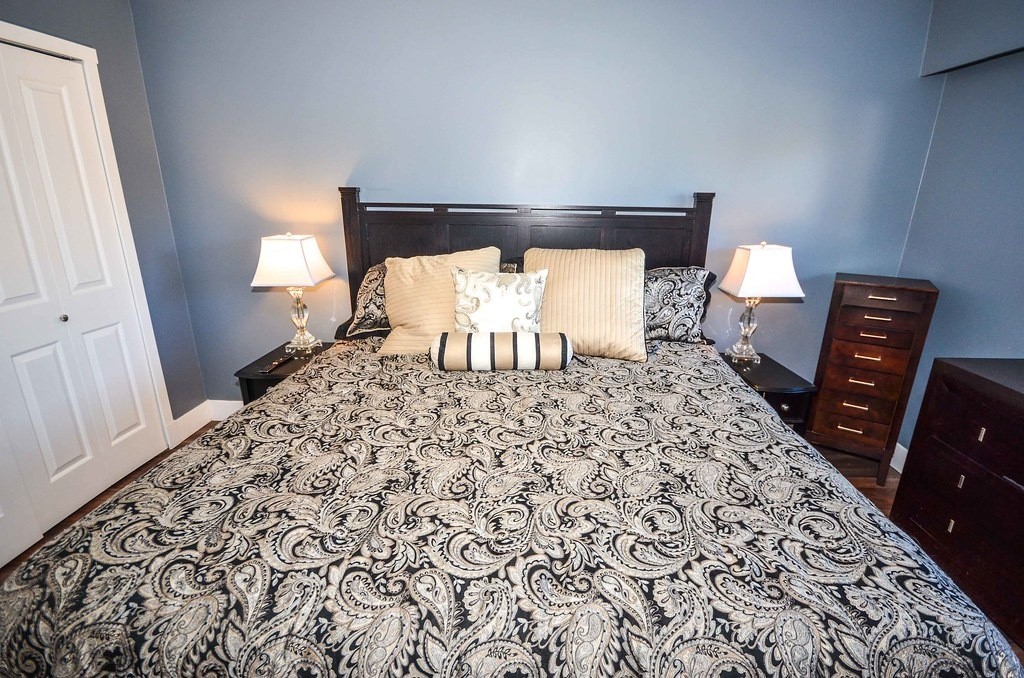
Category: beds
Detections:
[{"x1": 0, "y1": 188, "x2": 1024, "y2": 678}]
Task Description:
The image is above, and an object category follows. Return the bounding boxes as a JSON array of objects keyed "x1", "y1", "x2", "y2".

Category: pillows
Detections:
[
  {"x1": 374, "y1": 246, "x2": 500, "y2": 357},
  {"x1": 644, "y1": 265, "x2": 718, "y2": 345},
  {"x1": 524, "y1": 247, "x2": 647, "y2": 364},
  {"x1": 451, "y1": 265, "x2": 548, "y2": 334},
  {"x1": 431, "y1": 331, "x2": 574, "y2": 371},
  {"x1": 334, "y1": 257, "x2": 524, "y2": 341}
]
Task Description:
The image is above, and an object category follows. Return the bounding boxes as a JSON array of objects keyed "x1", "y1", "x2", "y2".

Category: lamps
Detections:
[
  {"x1": 718, "y1": 241, "x2": 805, "y2": 365},
  {"x1": 250, "y1": 232, "x2": 336, "y2": 353}
]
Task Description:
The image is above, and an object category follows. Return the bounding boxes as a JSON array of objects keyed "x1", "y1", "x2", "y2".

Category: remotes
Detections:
[{"x1": 259, "y1": 353, "x2": 294, "y2": 373}]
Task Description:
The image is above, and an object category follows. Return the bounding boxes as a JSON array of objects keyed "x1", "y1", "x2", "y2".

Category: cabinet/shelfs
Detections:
[
  {"x1": 805, "y1": 273, "x2": 939, "y2": 486},
  {"x1": 889, "y1": 358, "x2": 1024, "y2": 651}
]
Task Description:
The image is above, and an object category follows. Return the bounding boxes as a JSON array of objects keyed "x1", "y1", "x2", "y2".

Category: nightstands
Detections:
[
  {"x1": 719, "y1": 352, "x2": 818, "y2": 437},
  {"x1": 234, "y1": 342, "x2": 336, "y2": 407}
]
